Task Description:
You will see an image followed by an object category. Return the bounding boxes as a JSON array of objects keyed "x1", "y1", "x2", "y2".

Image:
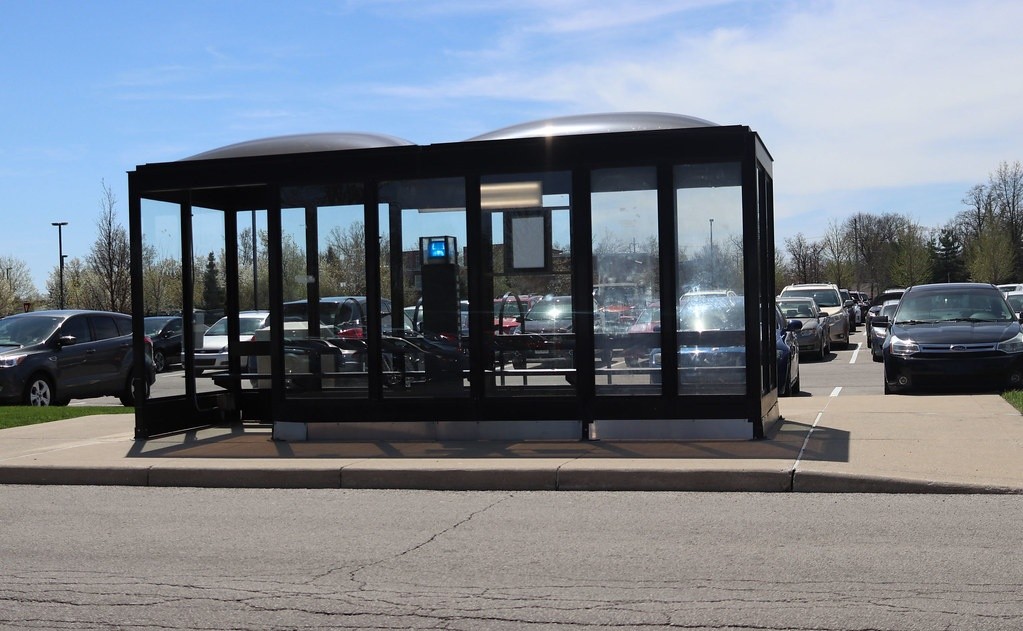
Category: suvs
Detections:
[
  {"x1": 247, "y1": 296, "x2": 421, "y2": 389},
  {"x1": 779, "y1": 283, "x2": 854, "y2": 349}
]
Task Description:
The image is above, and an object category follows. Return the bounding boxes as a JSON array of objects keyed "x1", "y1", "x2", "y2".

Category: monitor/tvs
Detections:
[{"x1": 428, "y1": 241, "x2": 448, "y2": 259}]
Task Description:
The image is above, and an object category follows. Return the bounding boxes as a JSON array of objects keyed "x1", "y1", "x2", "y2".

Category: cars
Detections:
[
  {"x1": 410, "y1": 280, "x2": 660, "y2": 362},
  {"x1": 511, "y1": 295, "x2": 612, "y2": 371},
  {"x1": 0, "y1": 309, "x2": 156, "y2": 407},
  {"x1": 193, "y1": 308, "x2": 272, "y2": 377},
  {"x1": 869, "y1": 299, "x2": 900, "y2": 362},
  {"x1": 648, "y1": 293, "x2": 803, "y2": 397},
  {"x1": 839, "y1": 282, "x2": 1023, "y2": 348},
  {"x1": 871, "y1": 281, "x2": 1023, "y2": 395},
  {"x1": 775, "y1": 294, "x2": 830, "y2": 361},
  {"x1": 145, "y1": 316, "x2": 211, "y2": 374}
]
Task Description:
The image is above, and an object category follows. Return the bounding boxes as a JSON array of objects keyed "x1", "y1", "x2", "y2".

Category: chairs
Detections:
[
  {"x1": 798, "y1": 305, "x2": 811, "y2": 316},
  {"x1": 913, "y1": 298, "x2": 935, "y2": 319},
  {"x1": 1009, "y1": 299, "x2": 1021, "y2": 311},
  {"x1": 967, "y1": 295, "x2": 994, "y2": 318}
]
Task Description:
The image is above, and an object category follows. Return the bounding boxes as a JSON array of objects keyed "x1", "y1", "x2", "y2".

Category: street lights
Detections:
[
  {"x1": 709, "y1": 219, "x2": 714, "y2": 291},
  {"x1": 52, "y1": 222, "x2": 69, "y2": 310}
]
